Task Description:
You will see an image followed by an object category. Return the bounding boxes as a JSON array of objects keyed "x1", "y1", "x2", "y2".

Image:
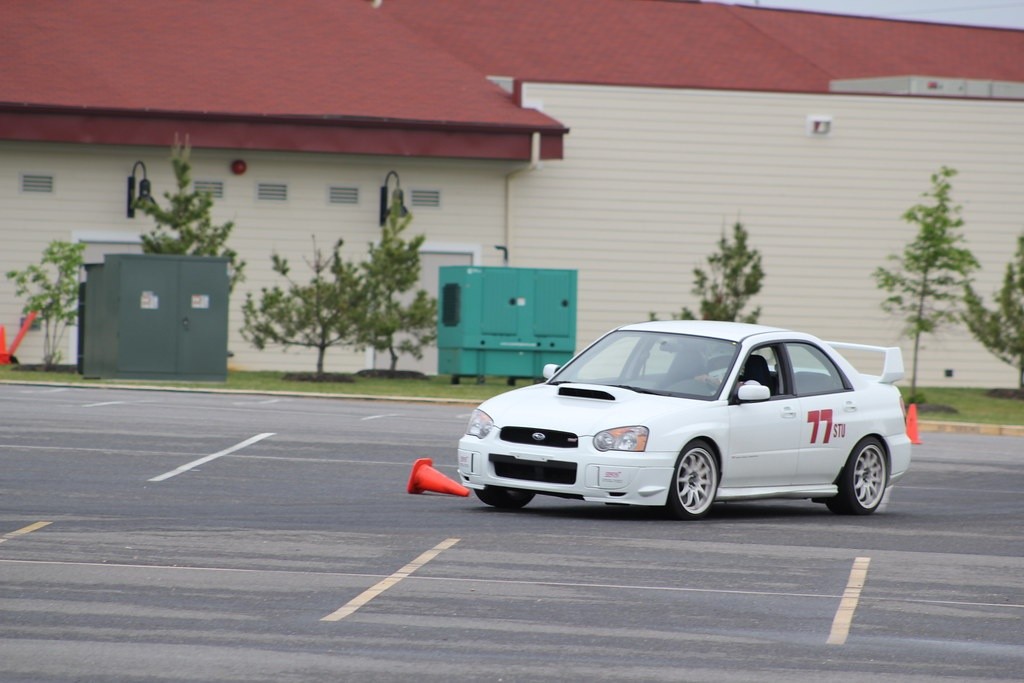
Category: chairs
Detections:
[
  {"x1": 668, "y1": 350, "x2": 704, "y2": 381},
  {"x1": 743, "y1": 356, "x2": 771, "y2": 397}
]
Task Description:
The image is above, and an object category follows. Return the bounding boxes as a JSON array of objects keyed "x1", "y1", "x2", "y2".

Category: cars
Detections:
[{"x1": 457, "y1": 321, "x2": 913, "y2": 520}]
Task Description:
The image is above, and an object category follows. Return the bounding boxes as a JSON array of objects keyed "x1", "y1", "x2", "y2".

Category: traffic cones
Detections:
[
  {"x1": 906, "y1": 403, "x2": 922, "y2": 444},
  {"x1": 407, "y1": 458, "x2": 471, "y2": 498}
]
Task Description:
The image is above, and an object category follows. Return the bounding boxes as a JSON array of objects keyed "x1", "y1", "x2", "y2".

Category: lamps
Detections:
[
  {"x1": 806, "y1": 115, "x2": 832, "y2": 137},
  {"x1": 380, "y1": 171, "x2": 408, "y2": 225},
  {"x1": 126, "y1": 162, "x2": 156, "y2": 218}
]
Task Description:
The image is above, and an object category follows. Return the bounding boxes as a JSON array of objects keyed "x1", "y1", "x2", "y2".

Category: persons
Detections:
[{"x1": 695, "y1": 368, "x2": 747, "y2": 400}]
